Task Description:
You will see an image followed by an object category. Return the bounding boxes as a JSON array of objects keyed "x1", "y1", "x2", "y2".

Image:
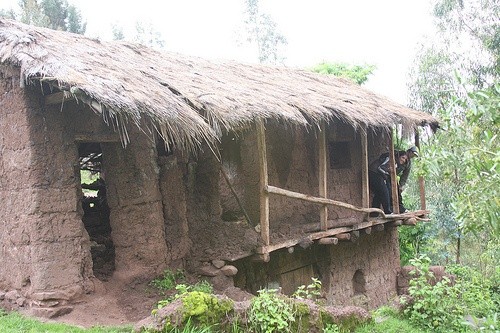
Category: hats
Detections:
[{"x1": 411, "y1": 146, "x2": 419, "y2": 157}]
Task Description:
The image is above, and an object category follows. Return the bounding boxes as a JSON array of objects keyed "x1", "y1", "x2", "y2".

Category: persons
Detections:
[
  {"x1": 386, "y1": 145, "x2": 420, "y2": 215},
  {"x1": 368, "y1": 150, "x2": 408, "y2": 218}
]
